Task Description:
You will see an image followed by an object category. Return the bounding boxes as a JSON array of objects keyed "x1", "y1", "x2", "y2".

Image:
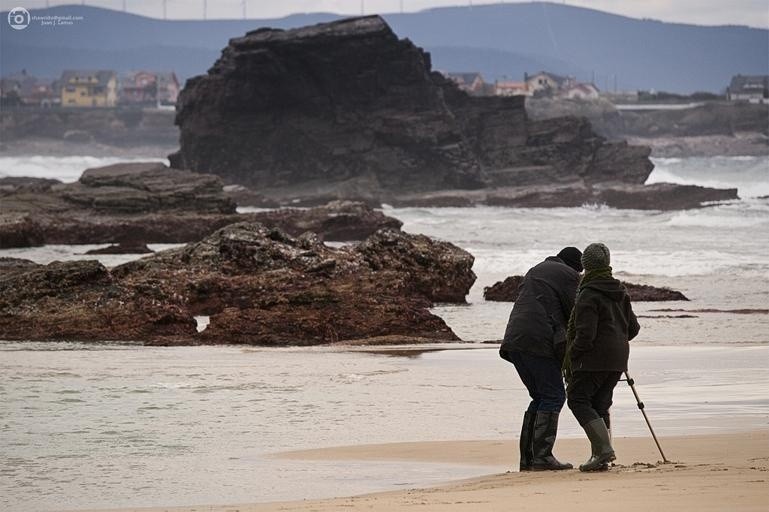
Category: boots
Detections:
[
  {"x1": 530, "y1": 408, "x2": 580, "y2": 471},
  {"x1": 518, "y1": 410, "x2": 535, "y2": 471},
  {"x1": 579, "y1": 415, "x2": 616, "y2": 473}
]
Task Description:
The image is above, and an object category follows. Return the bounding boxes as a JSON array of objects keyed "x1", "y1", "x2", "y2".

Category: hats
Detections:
[
  {"x1": 558, "y1": 246, "x2": 585, "y2": 274},
  {"x1": 581, "y1": 241, "x2": 612, "y2": 272}
]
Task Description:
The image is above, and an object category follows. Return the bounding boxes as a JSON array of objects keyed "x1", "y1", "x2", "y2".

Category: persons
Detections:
[
  {"x1": 498, "y1": 246, "x2": 584, "y2": 471},
  {"x1": 563, "y1": 243, "x2": 640, "y2": 473}
]
